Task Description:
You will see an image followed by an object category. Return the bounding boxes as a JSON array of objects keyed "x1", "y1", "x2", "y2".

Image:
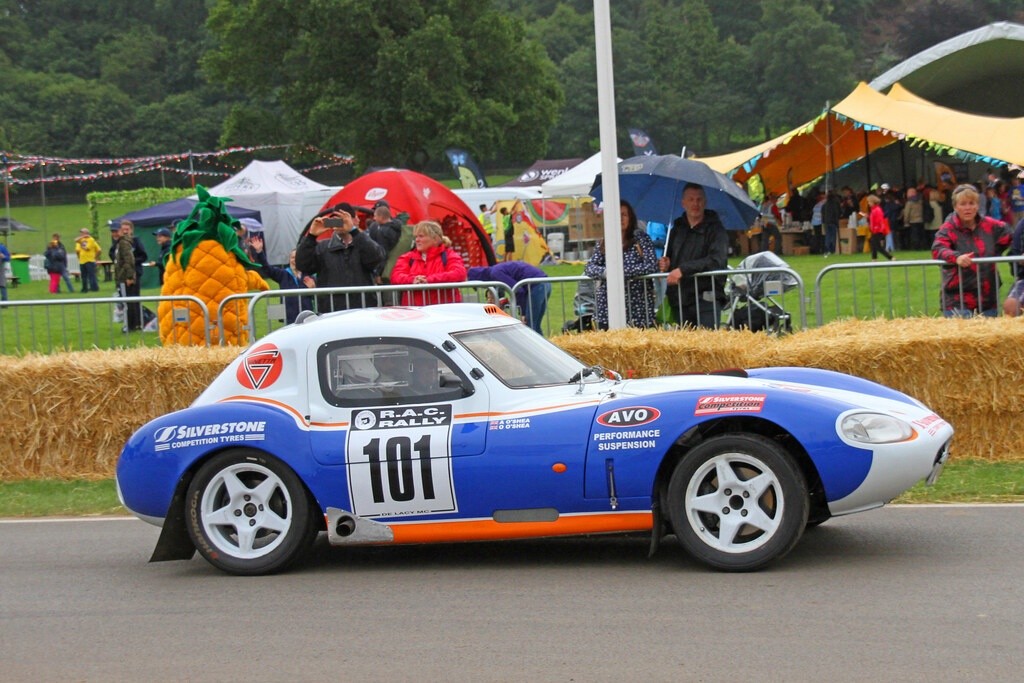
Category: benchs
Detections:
[
  {"x1": 4, "y1": 277, "x2": 20, "y2": 287},
  {"x1": 71, "y1": 270, "x2": 115, "y2": 282}
]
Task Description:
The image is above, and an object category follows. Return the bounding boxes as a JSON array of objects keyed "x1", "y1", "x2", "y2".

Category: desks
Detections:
[
  {"x1": 778, "y1": 231, "x2": 806, "y2": 255},
  {"x1": 96, "y1": 260, "x2": 114, "y2": 282},
  {"x1": 568, "y1": 238, "x2": 603, "y2": 260}
]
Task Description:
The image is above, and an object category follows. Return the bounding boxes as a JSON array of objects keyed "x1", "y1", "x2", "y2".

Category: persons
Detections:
[
  {"x1": 931, "y1": 184, "x2": 1014, "y2": 318},
  {"x1": 0, "y1": 166, "x2": 552, "y2": 337},
  {"x1": 726, "y1": 154, "x2": 1024, "y2": 317},
  {"x1": 584, "y1": 199, "x2": 658, "y2": 331},
  {"x1": 658, "y1": 182, "x2": 730, "y2": 331},
  {"x1": 371, "y1": 348, "x2": 461, "y2": 401},
  {"x1": 296, "y1": 203, "x2": 387, "y2": 313},
  {"x1": 389, "y1": 221, "x2": 467, "y2": 306}
]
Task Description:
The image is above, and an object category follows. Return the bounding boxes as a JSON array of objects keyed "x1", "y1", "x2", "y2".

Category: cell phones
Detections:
[{"x1": 322, "y1": 218, "x2": 343, "y2": 228}]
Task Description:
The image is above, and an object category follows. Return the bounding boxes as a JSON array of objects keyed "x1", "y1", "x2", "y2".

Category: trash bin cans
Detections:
[{"x1": 10, "y1": 254, "x2": 30, "y2": 283}]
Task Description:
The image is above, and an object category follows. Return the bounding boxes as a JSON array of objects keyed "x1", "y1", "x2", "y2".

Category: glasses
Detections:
[{"x1": 955, "y1": 186, "x2": 977, "y2": 195}]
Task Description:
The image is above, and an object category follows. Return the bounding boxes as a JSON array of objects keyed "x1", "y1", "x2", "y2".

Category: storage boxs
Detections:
[
  {"x1": 839, "y1": 228, "x2": 857, "y2": 253},
  {"x1": 569, "y1": 202, "x2": 603, "y2": 240},
  {"x1": 793, "y1": 246, "x2": 809, "y2": 256}
]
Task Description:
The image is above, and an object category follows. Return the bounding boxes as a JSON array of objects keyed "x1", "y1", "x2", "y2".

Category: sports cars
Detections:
[{"x1": 115, "y1": 301, "x2": 955, "y2": 577}]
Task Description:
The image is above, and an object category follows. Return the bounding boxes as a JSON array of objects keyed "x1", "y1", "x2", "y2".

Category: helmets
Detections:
[
  {"x1": 407, "y1": 349, "x2": 438, "y2": 395},
  {"x1": 369, "y1": 344, "x2": 409, "y2": 392}
]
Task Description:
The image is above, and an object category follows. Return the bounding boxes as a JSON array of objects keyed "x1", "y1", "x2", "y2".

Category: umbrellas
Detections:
[
  {"x1": 589, "y1": 145, "x2": 764, "y2": 288},
  {"x1": 540, "y1": 150, "x2": 625, "y2": 201},
  {"x1": 0, "y1": 217, "x2": 40, "y2": 253}
]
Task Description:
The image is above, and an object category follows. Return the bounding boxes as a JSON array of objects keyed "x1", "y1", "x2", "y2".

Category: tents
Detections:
[
  {"x1": 295, "y1": 169, "x2": 500, "y2": 315},
  {"x1": 494, "y1": 158, "x2": 585, "y2": 245},
  {"x1": 103, "y1": 197, "x2": 270, "y2": 289},
  {"x1": 450, "y1": 186, "x2": 579, "y2": 268},
  {"x1": 186, "y1": 159, "x2": 344, "y2": 268}
]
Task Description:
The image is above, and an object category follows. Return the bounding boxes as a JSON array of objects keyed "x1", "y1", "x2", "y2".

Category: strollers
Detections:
[{"x1": 724, "y1": 250, "x2": 799, "y2": 335}]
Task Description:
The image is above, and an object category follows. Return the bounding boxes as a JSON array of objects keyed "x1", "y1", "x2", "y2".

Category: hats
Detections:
[
  {"x1": 169, "y1": 218, "x2": 185, "y2": 229},
  {"x1": 152, "y1": 228, "x2": 171, "y2": 237},
  {"x1": 109, "y1": 222, "x2": 121, "y2": 230},
  {"x1": 373, "y1": 201, "x2": 388, "y2": 209}
]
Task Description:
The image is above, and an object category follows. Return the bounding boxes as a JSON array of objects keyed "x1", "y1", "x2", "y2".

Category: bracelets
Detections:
[{"x1": 349, "y1": 226, "x2": 359, "y2": 233}]
